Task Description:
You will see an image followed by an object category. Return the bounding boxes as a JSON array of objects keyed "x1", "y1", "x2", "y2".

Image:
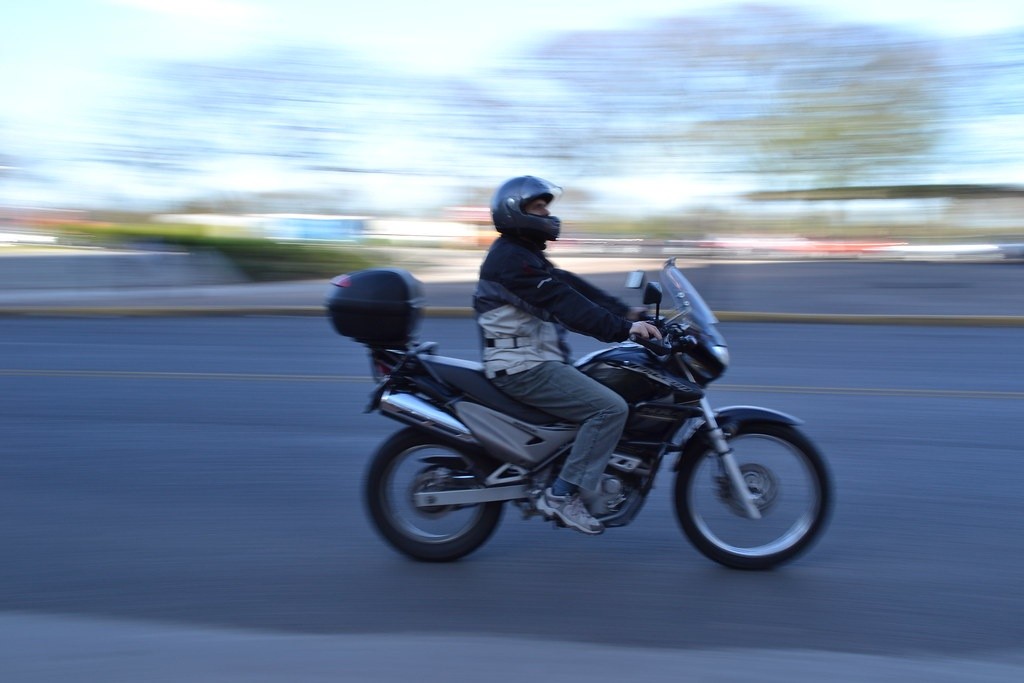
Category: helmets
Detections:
[{"x1": 491, "y1": 175, "x2": 561, "y2": 241}]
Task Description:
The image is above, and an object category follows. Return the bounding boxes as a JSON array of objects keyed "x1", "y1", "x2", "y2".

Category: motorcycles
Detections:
[{"x1": 325, "y1": 258, "x2": 836, "y2": 572}]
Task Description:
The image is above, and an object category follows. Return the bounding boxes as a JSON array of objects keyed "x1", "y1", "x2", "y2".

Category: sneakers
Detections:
[{"x1": 536, "y1": 487, "x2": 602, "y2": 535}]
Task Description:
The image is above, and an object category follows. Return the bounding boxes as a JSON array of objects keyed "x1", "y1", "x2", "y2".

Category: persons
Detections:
[{"x1": 472, "y1": 175, "x2": 663, "y2": 534}]
